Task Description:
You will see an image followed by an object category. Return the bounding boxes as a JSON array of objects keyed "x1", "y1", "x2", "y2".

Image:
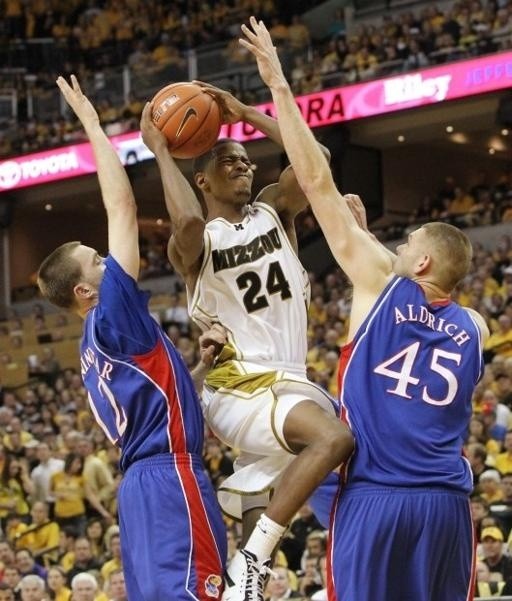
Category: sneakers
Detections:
[{"x1": 222, "y1": 549, "x2": 272, "y2": 601}]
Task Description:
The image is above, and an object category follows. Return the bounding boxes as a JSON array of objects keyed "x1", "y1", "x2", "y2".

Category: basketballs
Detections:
[{"x1": 151, "y1": 82, "x2": 223, "y2": 160}]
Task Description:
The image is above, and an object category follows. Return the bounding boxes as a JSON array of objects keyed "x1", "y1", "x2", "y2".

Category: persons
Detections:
[{"x1": 2, "y1": 3, "x2": 511, "y2": 601}]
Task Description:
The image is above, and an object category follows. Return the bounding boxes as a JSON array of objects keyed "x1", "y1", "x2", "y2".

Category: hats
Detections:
[{"x1": 480, "y1": 528, "x2": 503, "y2": 540}]
